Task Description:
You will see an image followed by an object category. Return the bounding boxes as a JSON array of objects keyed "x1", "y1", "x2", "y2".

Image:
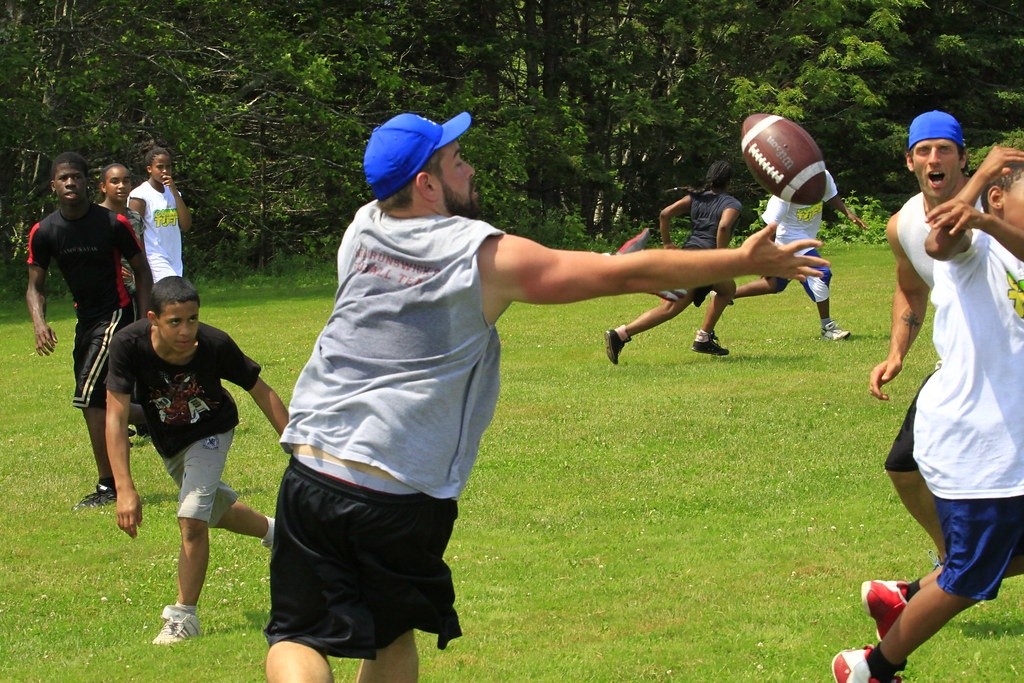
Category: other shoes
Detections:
[
  {"x1": 135, "y1": 425, "x2": 149, "y2": 438},
  {"x1": 710, "y1": 290, "x2": 734, "y2": 305}
]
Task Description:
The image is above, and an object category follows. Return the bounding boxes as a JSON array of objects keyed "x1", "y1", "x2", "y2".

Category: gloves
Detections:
[{"x1": 602, "y1": 228, "x2": 688, "y2": 302}]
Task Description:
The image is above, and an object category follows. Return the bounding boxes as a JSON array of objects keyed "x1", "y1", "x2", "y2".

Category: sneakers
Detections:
[
  {"x1": 832, "y1": 644, "x2": 902, "y2": 683},
  {"x1": 606, "y1": 324, "x2": 632, "y2": 364},
  {"x1": 71, "y1": 484, "x2": 116, "y2": 512},
  {"x1": 821, "y1": 320, "x2": 850, "y2": 341},
  {"x1": 692, "y1": 330, "x2": 729, "y2": 356},
  {"x1": 862, "y1": 581, "x2": 909, "y2": 641},
  {"x1": 151, "y1": 605, "x2": 200, "y2": 646}
]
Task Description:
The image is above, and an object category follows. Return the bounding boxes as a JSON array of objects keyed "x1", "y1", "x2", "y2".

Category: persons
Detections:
[
  {"x1": 127, "y1": 148, "x2": 192, "y2": 282},
  {"x1": 605, "y1": 161, "x2": 742, "y2": 363},
  {"x1": 94, "y1": 162, "x2": 147, "y2": 401},
  {"x1": 104, "y1": 276, "x2": 290, "y2": 646},
  {"x1": 832, "y1": 108, "x2": 1024, "y2": 683},
  {"x1": 26, "y1": 151, "x2": 147, "y2": 508},
  {"x1": 711, "y1": 169, "x2": 868, "y2": 341},
  {"x1": 265, "y1": 112, "x2": 831, "y2": 683}
]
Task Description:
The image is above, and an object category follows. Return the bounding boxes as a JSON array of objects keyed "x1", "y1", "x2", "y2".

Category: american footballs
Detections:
[{"x1": 741, "y1": 112, "x2": 827, "y2": 205}]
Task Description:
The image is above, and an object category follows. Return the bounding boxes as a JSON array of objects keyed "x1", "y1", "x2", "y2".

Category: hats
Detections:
[
  {"x1": 908, "y1": 109, "x2": 965, "y2": 151},
  {"x1": 363, "y1": 112, "x2": 472, "y2": 200}
]
